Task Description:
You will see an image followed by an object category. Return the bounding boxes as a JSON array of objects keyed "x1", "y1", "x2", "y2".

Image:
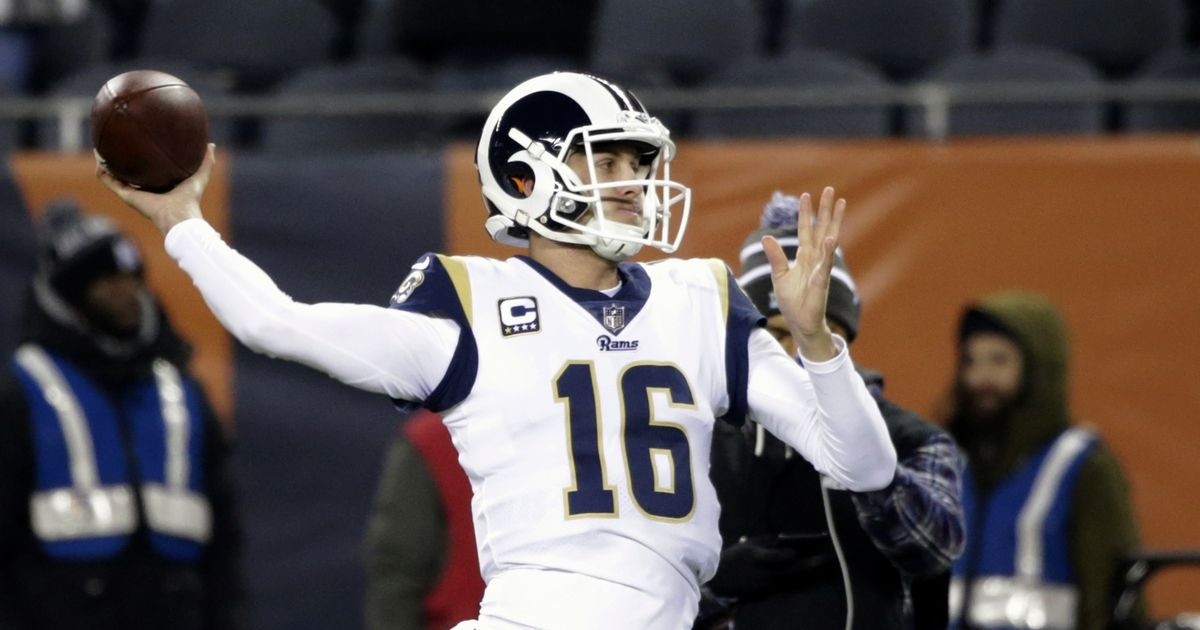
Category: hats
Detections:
[{"x1": 738, "y1": 191, "x2": 861, "y2": 342}]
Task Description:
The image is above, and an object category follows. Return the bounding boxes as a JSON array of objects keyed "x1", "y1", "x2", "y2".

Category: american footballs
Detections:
[{"x1": 90, "y1": 67, "x2": 209, "y2": 191}]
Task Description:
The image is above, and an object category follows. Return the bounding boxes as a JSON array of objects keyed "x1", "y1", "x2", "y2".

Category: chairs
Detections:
[{"x1": 0, "y1": 1, "x2": 1200, "y2": 151}]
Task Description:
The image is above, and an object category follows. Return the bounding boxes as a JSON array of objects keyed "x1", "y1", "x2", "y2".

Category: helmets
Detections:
[
  {"x1": 26, "y1": 217, "x2": 162, "y2": 366},
  {"x1": 475, "y1": 70, "x2": 693, "y2": 263}
]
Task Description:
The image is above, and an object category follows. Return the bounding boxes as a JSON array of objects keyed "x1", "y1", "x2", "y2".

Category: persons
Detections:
[
  {"x1": 94, "y1": 70, "x2": 897, "y2": 630},
  {"x1": 693, "y1": 192, "x2": 967, "y2": 630},
  {"x1": 0, "y1": 200, "x2": 252, "y2": 630},
  {"x1": 368, "y1": 411, "x2": 485, "y2": 630},
  {"x1": 938, "y1": 298, "x2": 1149, "y2": 630}
]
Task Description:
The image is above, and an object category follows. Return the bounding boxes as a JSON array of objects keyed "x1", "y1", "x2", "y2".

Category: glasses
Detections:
[{"x1": 766, "y1": 323, "x2": 792, "y2": 341}]
